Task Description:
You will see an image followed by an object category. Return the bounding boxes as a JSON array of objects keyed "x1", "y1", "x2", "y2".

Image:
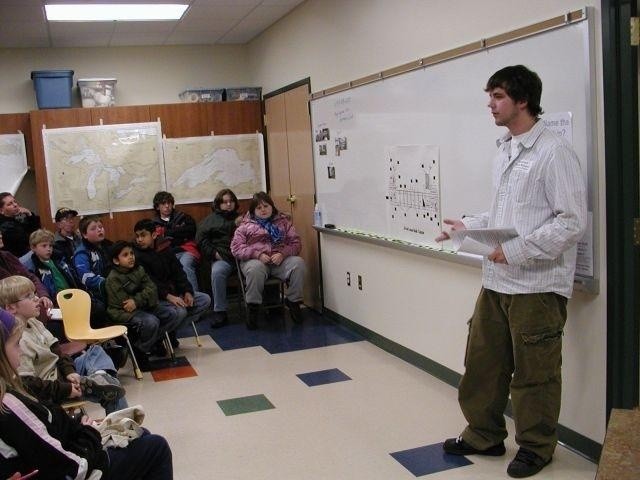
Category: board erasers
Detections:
[{"x1": 324, "y1": 223, "x2": 336, "y2": 229}]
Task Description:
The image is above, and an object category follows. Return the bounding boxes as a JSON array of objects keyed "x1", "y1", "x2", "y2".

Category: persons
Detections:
[
  {"x1": 434, "y1": 64, "x2": 588, "y2": 479},
  {"x1": 0, "y1": 275, "x2": 129, "y2": 419},
  {"x1": 195, "y1": 189, "x2": 268, "y2": 330},
  {"x1": 0, "y1": 192, "x2": 211, "y2": 371},
  {"x1": 230, "y1": 191, "x2": 305, "y2": 332},
  {"x1": 0, "y1": 309, "x2": 174, "y2": 480}
]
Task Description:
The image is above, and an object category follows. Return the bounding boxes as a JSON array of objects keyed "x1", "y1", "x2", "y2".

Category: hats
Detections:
[{"x1": 54, "y1": 206, "x2": 78, "y2": 223}]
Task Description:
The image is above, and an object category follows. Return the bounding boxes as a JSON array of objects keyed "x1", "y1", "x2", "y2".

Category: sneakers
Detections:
[
  {"x1": 285, "y1": 297, "x2": 305, "y2": 323},
  {"x1": 210, "y1": 311, "x2": 229, "y2": 329},
  {"x1": 442, "y1": 434, "x2": 508, "y2": 457},
  {"x1": 131, "y1": 329, "x2": 182, "y2": 370},
  {"x1": 506, "y1": 442, "x2": 553, "y2": 479},
  {"x1": 79, "y1": 370, "x2": 126, "y2": 404},
  {"x1": 246, "y1": 302, "x2": 261, "y2": 330}
]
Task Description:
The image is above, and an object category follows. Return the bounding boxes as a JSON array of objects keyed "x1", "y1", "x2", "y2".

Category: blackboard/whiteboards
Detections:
[{"x1": 308, "y1": 5, "x2": 601, "y2": 294}]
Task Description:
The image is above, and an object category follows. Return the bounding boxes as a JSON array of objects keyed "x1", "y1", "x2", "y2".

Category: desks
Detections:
[{"x1": 592, "y1": 404, "x2": 640, "y2": 479}]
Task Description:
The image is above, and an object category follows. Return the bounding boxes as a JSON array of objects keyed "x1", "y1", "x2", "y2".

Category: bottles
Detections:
[{"x1": 313, "y1": 203, "x2": 323, "y2": 227}]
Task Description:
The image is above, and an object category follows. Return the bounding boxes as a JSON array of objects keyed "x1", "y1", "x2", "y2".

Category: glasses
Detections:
[{"x1": 0, "y1": 288, "x2": 38, "y2": 305}]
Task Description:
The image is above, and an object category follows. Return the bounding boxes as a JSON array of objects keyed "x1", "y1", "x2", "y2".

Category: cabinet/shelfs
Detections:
[{"x1": 28, "y1": 98, "x2": 267, "y2": 251}]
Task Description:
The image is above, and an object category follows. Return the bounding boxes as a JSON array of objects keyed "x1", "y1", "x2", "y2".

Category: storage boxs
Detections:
[
  {"x1": 74, "y1": 76, "x2": 121, "y2": 110},
  {"x1": 30, "y1": 69, "x2": 76, "y2": 109},
  {"x1": 223, "y1": 83, "x2": 263, "y2": 102},
  {"x1": 178, "y1": 85, "x2": 225, "y2": 106}
]
